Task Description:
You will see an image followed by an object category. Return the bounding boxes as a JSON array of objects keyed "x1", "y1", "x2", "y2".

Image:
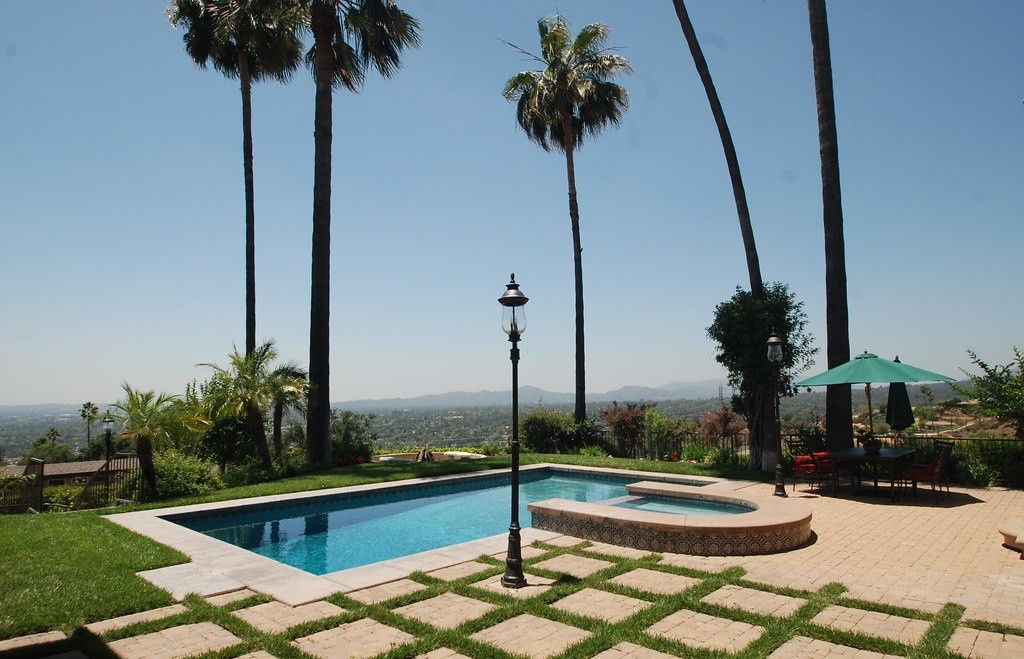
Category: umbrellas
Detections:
[
  {"x1": 884, "y1": 354, "x2": 917, "y2": 450},
  {"x1": 795, "y1": 348, "x2": 957, "y2": 454}
]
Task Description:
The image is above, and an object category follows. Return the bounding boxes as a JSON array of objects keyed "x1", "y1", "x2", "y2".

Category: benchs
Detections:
[
  {"x1": 444, "y1": 451, "x2": 471, "y2": 460},
  {"x1": 379, "y1": 457, "x2": 413, "y2": 464}
]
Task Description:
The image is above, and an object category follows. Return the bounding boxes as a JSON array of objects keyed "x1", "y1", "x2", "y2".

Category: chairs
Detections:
[
  {"x1": 897, "y1": 439, "x2": 955, "y2": 496},
  {"x1": 788, "y1": 434, "x2": 839, "y2": 496}
]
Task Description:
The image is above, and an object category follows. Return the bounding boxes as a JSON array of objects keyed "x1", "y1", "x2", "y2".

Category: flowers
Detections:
[{"x1": 858, "y1": 429, "x2": 882, "y2": 446}]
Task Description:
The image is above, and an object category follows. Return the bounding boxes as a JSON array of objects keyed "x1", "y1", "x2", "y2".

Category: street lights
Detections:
[
  {"x1": 498, "y1": 272, "x2": 530, "y2": 589},
  {"x1": 101, "y1": 410, "x2": 115, "y2": 505},
  {"x1": 765, "y1": 325, "x2": 788, "y2": 499}
]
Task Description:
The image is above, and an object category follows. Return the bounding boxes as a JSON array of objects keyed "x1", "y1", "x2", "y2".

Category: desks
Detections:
[{"x1": 830, "y1": 448, "x2": 917, "y2": 503}]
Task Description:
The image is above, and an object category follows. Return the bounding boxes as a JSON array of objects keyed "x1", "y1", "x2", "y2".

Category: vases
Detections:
[{"x1": 864, "y1": 446, "x2": 880, "y2": 454}]
